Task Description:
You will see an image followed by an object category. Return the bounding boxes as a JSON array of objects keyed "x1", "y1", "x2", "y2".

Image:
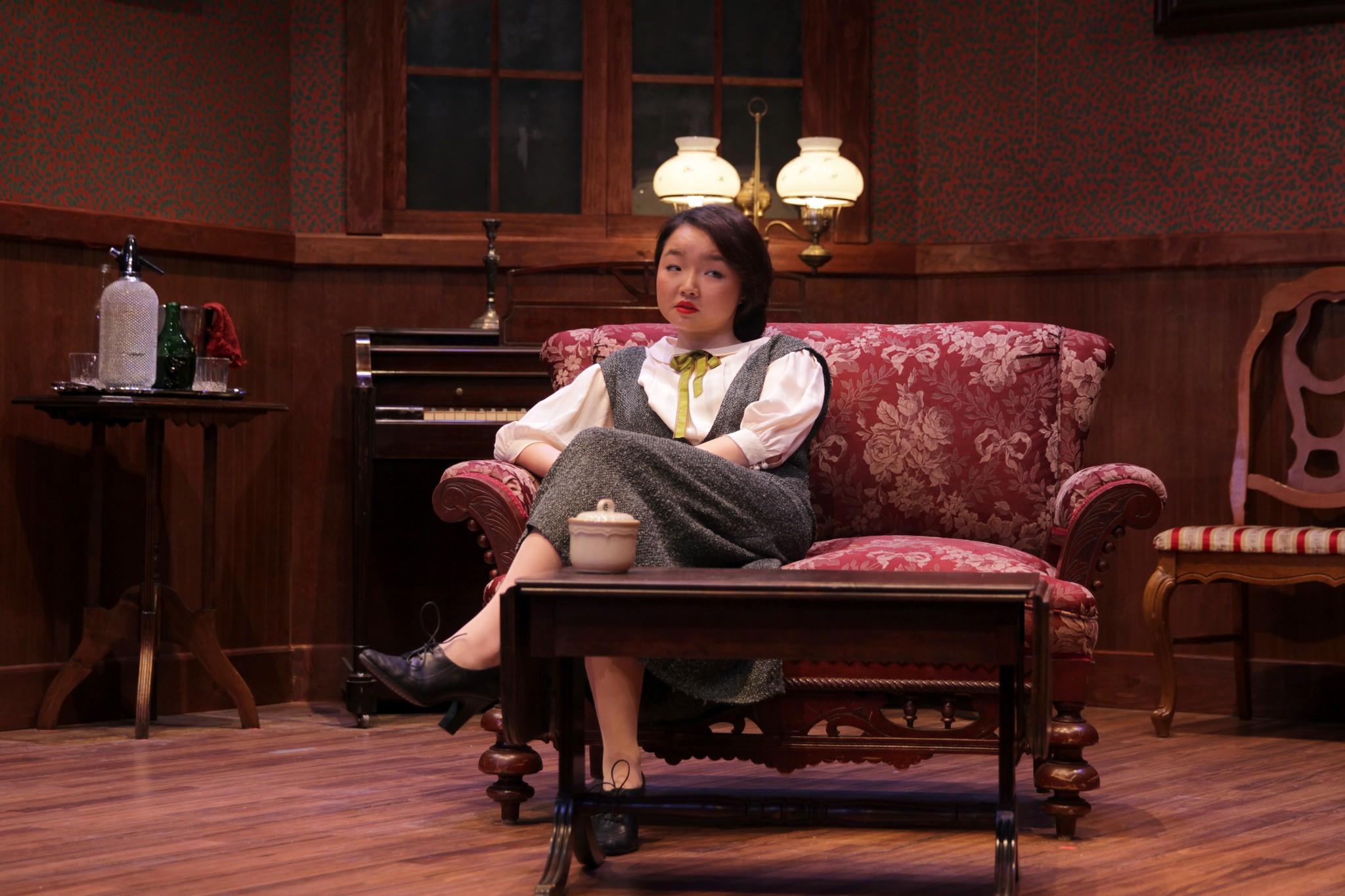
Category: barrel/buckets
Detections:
[{"x1": 159, "y1": 304, "x2": 212, "y2": 376}]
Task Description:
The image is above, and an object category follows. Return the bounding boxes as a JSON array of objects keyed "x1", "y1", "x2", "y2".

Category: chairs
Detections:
[{"x1": 1143, "y1": 266, "x2": 1345, "y2": 739}]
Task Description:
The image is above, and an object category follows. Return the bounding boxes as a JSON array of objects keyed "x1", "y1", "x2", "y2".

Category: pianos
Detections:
[{"x1": 339, "y1": 323, "x2": 550, "y2": 726}]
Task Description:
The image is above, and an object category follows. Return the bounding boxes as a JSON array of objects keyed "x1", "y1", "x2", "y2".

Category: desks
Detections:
[
  {"x1": 10, "y1": 393, "x2": 292, "y2": 740},
  {"x1": 499, "y1": 566, "x2": 1055, "y2": 896}
]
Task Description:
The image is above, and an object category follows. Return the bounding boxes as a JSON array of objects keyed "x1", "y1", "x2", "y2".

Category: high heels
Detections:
[
  {"x1": 584, "y1": 759, "x2": 649, "y2": 856},
  {"x1": 356, "y1": 600, "x2": 504, "y2": 736}
]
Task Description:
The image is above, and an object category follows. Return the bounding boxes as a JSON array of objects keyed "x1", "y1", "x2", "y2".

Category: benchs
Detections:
[{"x1": 430, "y1": 320, "x2": 1169, "y2": 847}]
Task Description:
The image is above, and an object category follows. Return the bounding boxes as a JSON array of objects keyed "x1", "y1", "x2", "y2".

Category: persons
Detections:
[{"x1": 359, "y1": 200, "x2": 833, "y2": 857}]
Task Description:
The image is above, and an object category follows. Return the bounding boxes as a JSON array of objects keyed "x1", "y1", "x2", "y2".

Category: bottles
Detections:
[
  {"x1": 98, "y1": 234, "x2": 159, "y2": 389},
  {"x1": 91, "y1": 263, "x2": 111, "y2": 350},
  {"x1": 153, "y1": 302, "x2": 196, "y2": 392}
]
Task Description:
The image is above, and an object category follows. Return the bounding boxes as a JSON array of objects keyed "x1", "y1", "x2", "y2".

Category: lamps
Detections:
[{"x1": 652, "y1": 96, "x2": 864, "y2": 275}]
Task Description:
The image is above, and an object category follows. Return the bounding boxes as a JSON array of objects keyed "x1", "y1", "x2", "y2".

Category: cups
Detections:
[
  {"x1": 70, "y1": 352, "x2": 98, "y2": 387},
  {"x1": 569, "y1": 499, "x2": 641, "y2": 574},
  {"x1": 192, "y1": 357, "x2": 228, "y2": 393}
]
